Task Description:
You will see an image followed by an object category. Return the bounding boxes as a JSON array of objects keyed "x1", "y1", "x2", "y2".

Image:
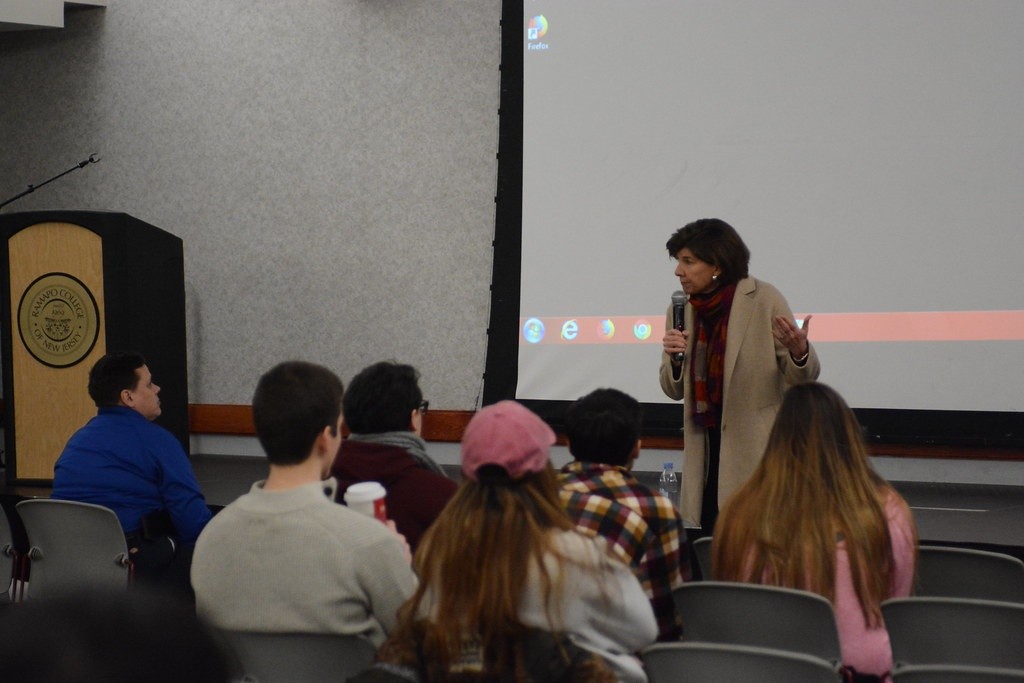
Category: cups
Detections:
[{"x1": 344, "y1": 481, "x2": 387, "y2": 530}]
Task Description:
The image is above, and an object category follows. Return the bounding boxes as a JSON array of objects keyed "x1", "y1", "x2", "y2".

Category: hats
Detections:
[{"x1": 457, "y1": 400, "x2": 557, "y2": 478}]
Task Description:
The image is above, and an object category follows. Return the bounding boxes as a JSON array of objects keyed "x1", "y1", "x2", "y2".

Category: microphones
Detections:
[{"x1": 671, "y1": 288, "x2": 688, "y2": 360}]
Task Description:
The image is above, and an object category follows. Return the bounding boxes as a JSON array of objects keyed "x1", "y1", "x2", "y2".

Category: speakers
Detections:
[{"x1": 0, "y1": 205, "x2": 188, "y2": 493}]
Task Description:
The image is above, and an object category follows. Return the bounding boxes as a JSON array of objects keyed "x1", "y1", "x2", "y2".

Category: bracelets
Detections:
[{"x1": 794, "y1": 353, "x2": 809, "y2": 365}]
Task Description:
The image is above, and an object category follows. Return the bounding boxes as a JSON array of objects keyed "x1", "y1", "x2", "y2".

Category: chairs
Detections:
[
  {"x1": 889, "y1": 661, "x2": 1024, "y2": 683},
  {"x1": 16, "y1": 497, "x2": 139, "y2": 615},
  {"x1": 216, "y1": 622, "x2": 373, "y2": 682},
  {"x1": 880, "y1": 595, "x2": 1023, "y2": 670},
  {"x1": 639, "y1": 641, "x2": 855, "y2": 683},
  {"x1": 0, "y1": 503, "x2": 22, "y2": 608},
  {"x1": 911, "y1": 544, "x2": 1024, "y2": 609},
  {"x1": 670, "y1": 580, "x2": 891, "y2": 682}
]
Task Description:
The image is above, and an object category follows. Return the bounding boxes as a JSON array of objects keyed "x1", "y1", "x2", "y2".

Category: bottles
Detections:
[{"x1": 658, "y1": 463, "x2": 677, "y2": 501}]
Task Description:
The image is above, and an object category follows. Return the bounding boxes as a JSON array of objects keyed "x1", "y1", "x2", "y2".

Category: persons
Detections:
[
  {"x1": 190, "y1": 357, "x2": 423, "y2": 683},
  {"x1": 659, "y1": 217, "x2": 820, "y2": 580},
  {"x1": 328, "y1": 357, "x2": 461, "y2": 553},
  {"x1": 50, "y1": 350, "x2": 215, "y2": 608},
  {"x1": 549, "y1": 388, "x2": 704, "y2": 645},
  {"x1": 393, "y1": 400, "x2": 661, "y2": 683},
  {"x1": 710, "y1": 377, "x2": 915, "y2": 679}
]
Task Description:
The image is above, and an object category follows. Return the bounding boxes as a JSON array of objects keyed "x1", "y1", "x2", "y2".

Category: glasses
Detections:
[{"x1": 415, "y1": 400, "x2": 431, "y2": 414}]
